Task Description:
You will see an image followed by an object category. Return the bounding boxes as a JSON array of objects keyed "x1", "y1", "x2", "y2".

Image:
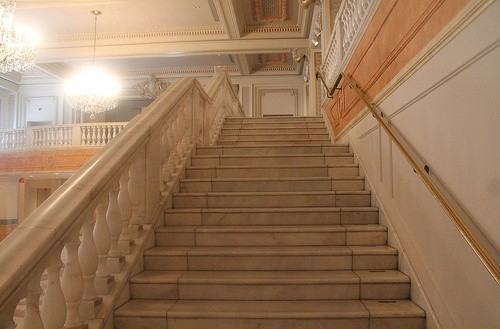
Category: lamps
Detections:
[
  {"x1": 0, "y1": 0, "x2": 40, "y2": 73},
  {"x1": 63, "y1": 10, "x2": 119, "y2": 120},
  {"x1": 314, "y1": 28, "x2": 321, "y2": 37},
  {"x1": 136, "y1": 74, "x2": 170, "y2": 99},
  {"x1": 311, "y1": 38, "x2": 319, "y2": 46}
]
